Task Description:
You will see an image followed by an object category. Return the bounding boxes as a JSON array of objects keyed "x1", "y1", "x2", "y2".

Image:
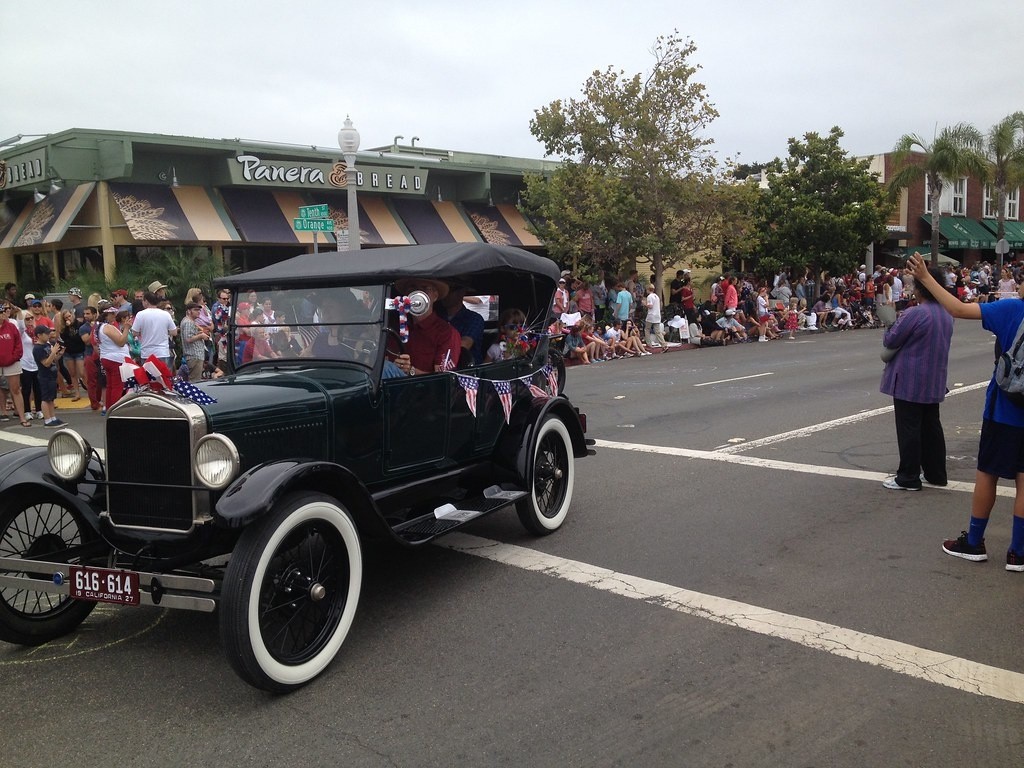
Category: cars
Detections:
[{"x1": 0, "y1": 242, "x2": 596, "y2": 694}]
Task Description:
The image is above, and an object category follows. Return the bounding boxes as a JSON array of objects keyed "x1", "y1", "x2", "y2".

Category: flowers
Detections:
[{"x1": 501, "y1": 305, "x2": 550, "y2": 361}]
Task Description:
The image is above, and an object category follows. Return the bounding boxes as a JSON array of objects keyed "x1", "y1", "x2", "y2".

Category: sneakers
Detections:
[
  {"x1": 943, "y1": 530, "x2": 990, "y2": 561},
  {"x1": 1006, "y1": 549, "x2": 1024, "y2": 572}
]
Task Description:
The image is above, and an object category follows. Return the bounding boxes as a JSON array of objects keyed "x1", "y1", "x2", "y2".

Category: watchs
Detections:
[{"x1": 409, "y1": 366, "x2": 417, "y2": 377}]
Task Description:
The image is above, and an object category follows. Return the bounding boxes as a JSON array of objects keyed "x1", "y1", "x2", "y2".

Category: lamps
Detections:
[
  {"x1": 168, "y1": 167, "x2": 180, "y2": 189},
  {"x1": 49, "y1": 178, "x2": 61, "y2": 196},
  {"x1": 514, "y1": 193, "x2": 522, "y2": 208},
  {"x1": 434, "y1": 186, "x2": 444, "y2": 204},
  {"x1": 32, "y1": 187, "x2": 47, "y2": 205},
  {"x1": 486, "y1": 195, "x2": 495, "y2": 207}
]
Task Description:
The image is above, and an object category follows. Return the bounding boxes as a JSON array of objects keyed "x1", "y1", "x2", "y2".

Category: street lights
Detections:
[{"x1": 337, "y1": 113, "x2": 362, "y2": 250}]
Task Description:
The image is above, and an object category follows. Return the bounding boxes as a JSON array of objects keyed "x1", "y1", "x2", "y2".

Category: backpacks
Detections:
[{"x1": 995, "y1": 315, "x2": 1024, "y2": 396}]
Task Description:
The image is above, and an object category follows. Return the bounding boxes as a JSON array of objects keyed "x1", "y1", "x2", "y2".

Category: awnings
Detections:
[
  {"x1": 108, "y1": 181, "x2": 552, "y2": 246},
  {"x1": 981, "y1": 219, "x2": 1024, "y2": 249},
  {"x1": 923, "y1": 213, "x2": 998, "y2": 250},
  {"x1": 0, "y1": 181, "x2": 97, "y2": 250}
]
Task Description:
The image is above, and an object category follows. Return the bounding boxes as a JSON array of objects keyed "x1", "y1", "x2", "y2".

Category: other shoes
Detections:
[
  {"x1": 920, "y1": 473, "x2": 936, "y2": 484},
  {"x1": 582, "y1": 323, "x2": 838, "y2": 365},
  {"x1": 882, "y1": 475, "x2": 921, "y2": 491},
  {"x1": 0, "y1": 392, "x2": 81, "y2": 429}
]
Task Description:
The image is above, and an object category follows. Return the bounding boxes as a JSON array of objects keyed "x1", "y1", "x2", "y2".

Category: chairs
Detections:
[{"x1": 481, "y1": 321, "x2": 501, "y2": 356}]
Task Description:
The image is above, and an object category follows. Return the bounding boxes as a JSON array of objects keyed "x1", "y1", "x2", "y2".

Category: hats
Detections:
[
  {"x1": 25, "y1": 294, "x2": 35, "y2": 300},
  {"x1": 102, "y1": 307, "x2": 120, "y2": 314},
  {"x1": 558, "y1": 278, "x2": 566, "y2": 283},
  {"x1": 187, "y1": 303, "x2": 202, "y2": 309},
  {"x1": 35, "y1": 325, "x2": 56, "y2": 335},
  {"x1": 442, "y1": 274, "x2": 476, "y2": 294},
  {"x1": 683, "y1": 268, "x2": 691, "y2": 275},
  {"x1": 69, "y1": 288, "x2": 83, "y2": 299},
  {"x1": 148, "y1": 281, "x2": 167, "y2": 294},
  {"x1": 31, "y1": 299, "x2": 42, "y2": 307},
  {"x1": 394, "y1": 276, "x2": 448, "y2": 302},
  {"x1": 112, "y1": 289, "x2": 128, "y2": 298}
]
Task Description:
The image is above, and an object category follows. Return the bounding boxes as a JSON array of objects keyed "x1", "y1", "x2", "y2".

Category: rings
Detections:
[{"x1": 400, "y1": 364, "x2": 403, "y2": 368}]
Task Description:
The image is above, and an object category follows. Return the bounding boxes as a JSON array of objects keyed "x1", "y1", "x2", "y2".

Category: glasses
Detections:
[
  {"x1": 0, "y1": 310, "x2": 7, "y2": 314},
  {"x1": 33, "y1": 304, "x2": 42, "y2": 308},
  {"x1": 26, "y1": 316, "x2": 34, "y2": 321},
  {"x1": 559, "y1": 282, "x2": 565, "y2": 284},
  {"x1": 164, "y1": 308, "x2": 173, "y2": 310},
  {"x1": 4, "y1": 307, "x2": 12, "y2": 311},
  {"x1": 220, "y1": 297, "x2": 229, "y2": 301}
]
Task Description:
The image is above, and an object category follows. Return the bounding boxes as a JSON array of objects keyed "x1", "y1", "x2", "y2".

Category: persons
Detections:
[
  {"x1": 297, "y1": 289, "x2": 376, "y2": 367},
  {"x1": 434, "y1": 276, "x2": 490, "y2": 369},
  {"x1": 484, "y1": 308, "x2": 533, "y2": 363},
  {"x1": 545, "y1": 258, "x2": 1024, "y2": 366},
  {"x1": 379, "y1": 277, "x2": 462, "y2": 379},
  {"x1": 0, "y1": 280, "x2": 298, "y2": 429},
  {"x1": 906, "y1": 250, "x2": 1024, "y2": 573},
  {"x1": 880, "y1": 263, "x2": 956, "y2": 491}
]
